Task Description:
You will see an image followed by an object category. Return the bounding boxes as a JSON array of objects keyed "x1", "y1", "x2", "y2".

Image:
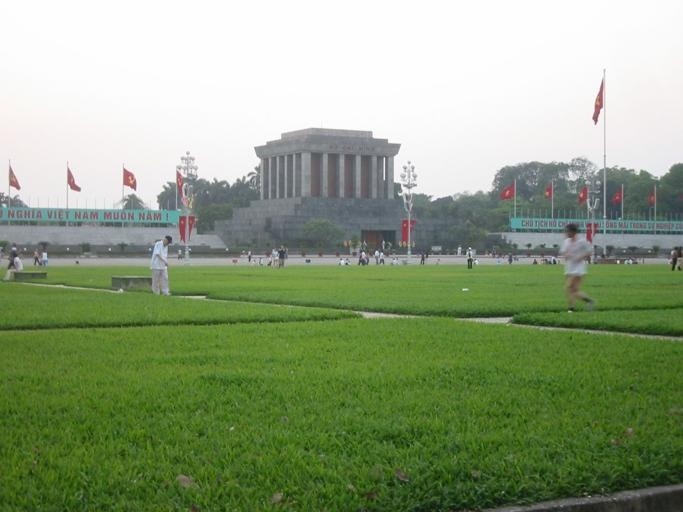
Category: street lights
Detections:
[
  {"x1": 585, "y1": 181, "x2": 601, "y2": 241},
  {"x1": 400, "y1": 161, "x2": 417, "y2": 255},
  {"x1": 178, "y1": 151, "x2": 198, "y2": 260}
]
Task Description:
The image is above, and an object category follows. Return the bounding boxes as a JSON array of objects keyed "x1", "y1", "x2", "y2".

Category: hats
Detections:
[{"x1": 165, "y1": 236, "x2": 172, "y2": 243}]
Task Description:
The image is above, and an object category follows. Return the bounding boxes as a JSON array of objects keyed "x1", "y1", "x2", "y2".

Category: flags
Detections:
[
  {"x1": 591, "y1": 80, "x2": 603, "y2": 126},
  {"x1": 499, "y1": 182, "x2": 514, "y2": 200},
  {"x1": 177, "y1": 170, "x2": 184, "y2": 193},
  {"x1": 189, "y1": 215, "x2": 195, "y2": 242},
  {"x1": 178, "y1": 216, "x2": 187, "y2": 242},
  {"x1": 9, "y1": 164, "x2": 21, "y2": 190},
  {"x1": 543, "y1": 183, "x2": 552, "y2": 200},
  {"x1": 123, "y1": 167, "x2": 136, "y2": 192},
  {"x1": 399, "y1": 219, "x2": 416, "y2": 243},
  {"x1": 612, "y1": 188, "x2": 622, "y2": 206},
  {"x1": 67, "y1": 166, "x2": 80, "y2": 192},
  {"x1": 578, "y1": 187, "x2": 587, "y2": 204},
  {"x1": 647, "y1": 189, "x2": 654, "y2": 208}
]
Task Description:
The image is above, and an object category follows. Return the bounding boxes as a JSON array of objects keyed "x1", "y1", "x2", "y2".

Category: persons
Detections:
[
  {"x1": 242, "y1": 244, "x2": 289, "y2": 268},
  {"x1": 461, "y1": 244, "x2": 682, "y2": 271},
  {"x1": 335, "y1": 238, "x2": 429, "y2": 266},
  {"x1": 150, "y1": 235, "x2": 172, "y2": 295},
  {"x1": 559, "y1": 223, "x2": 595, "y2": 312},
  {"x1": 0, "y1": 243, "x2": 49, "y2": 282}
]
